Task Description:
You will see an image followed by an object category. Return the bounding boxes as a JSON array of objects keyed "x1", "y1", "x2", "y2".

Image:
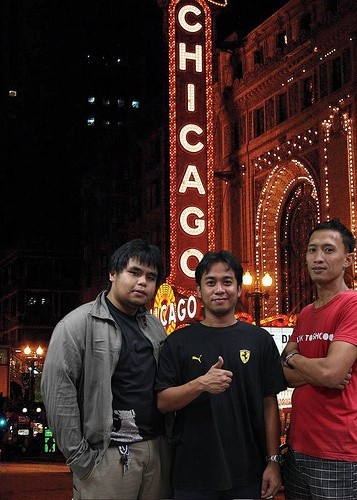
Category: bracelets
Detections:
[{"x1": 285, "y1": 351, "x2": 301, "y2": 366}]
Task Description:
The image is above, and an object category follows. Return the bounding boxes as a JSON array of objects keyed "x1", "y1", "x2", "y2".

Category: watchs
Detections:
[{"x1": 267, "y1": 454, "x2": 283, "y2": 463}]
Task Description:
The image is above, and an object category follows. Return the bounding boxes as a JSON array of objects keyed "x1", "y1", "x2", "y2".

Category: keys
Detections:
[{"x1": 118, "y1": 444, "x2": 131, "y2": 476}]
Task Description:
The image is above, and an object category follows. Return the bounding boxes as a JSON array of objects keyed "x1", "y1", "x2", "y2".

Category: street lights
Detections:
[
  {"x1": 243, "y1": 269, "x2": 274, "y2": 329},
  {"x1": 23, "y1": 344, "x2": 44, "y2": 403}
]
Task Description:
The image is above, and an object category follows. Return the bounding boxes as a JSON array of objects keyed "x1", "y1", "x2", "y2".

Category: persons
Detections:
[
  {"x1": 278, "y1": 219, "x2": 357, "y2": 500},
  {"x1": 158, "y1": 250, "x2": 288, "y2": 500},
  {"x1": 40, "y1": 237, "x2": 169, "y2": 499}
]
